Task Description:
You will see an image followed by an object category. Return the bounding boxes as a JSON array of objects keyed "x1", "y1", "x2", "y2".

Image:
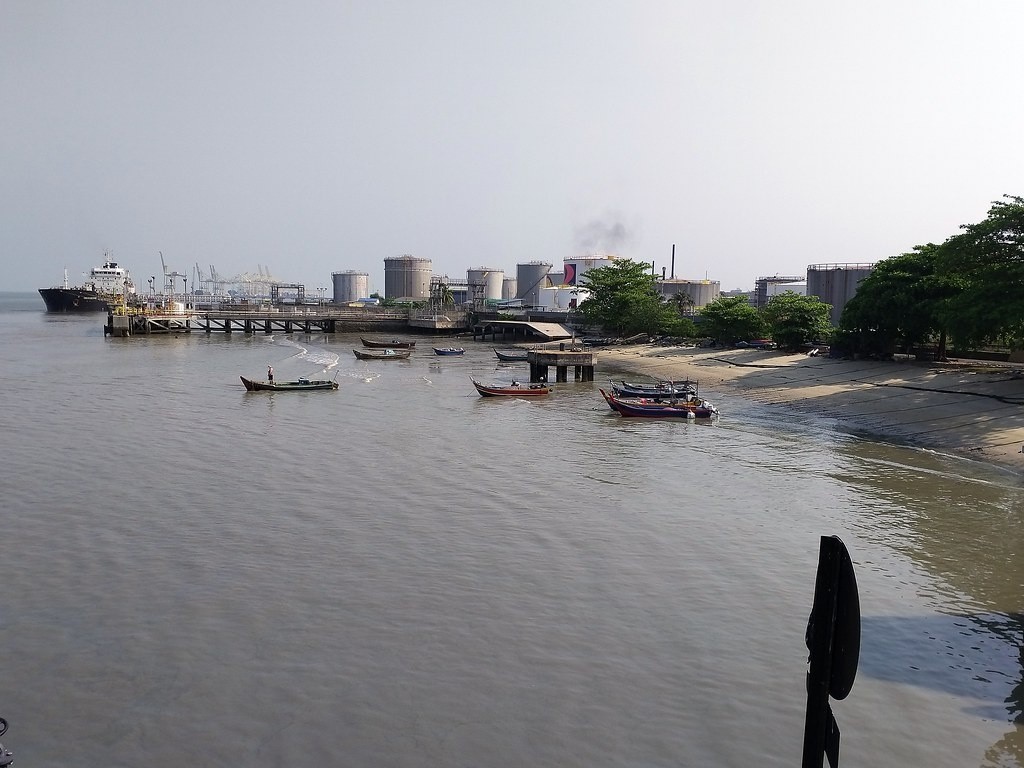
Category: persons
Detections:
[{"x1": 267, "y1": 365, "x2": 273, "y2": 384}]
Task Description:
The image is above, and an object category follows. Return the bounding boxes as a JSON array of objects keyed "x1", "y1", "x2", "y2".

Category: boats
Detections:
[
  {"x1": 469, "y1": 377, "x2": 549, "y2": 397},
  {"x1": 582, "y1": 338, "x2": 612, "y2": 347},
  {"x1": 240, "y1": 369, "x2": 339, "y2": 390},
  {"x1": 353, "y1": 349, "x2": 410, "y2": 359},
  {"x1": 599, "y1": 379, "x2": 714, "y2": 419},
  {"x1": 360, "y1": 337, "x2": 416, "y2": 347},
  {"x1": 432, "y1": 348, "x2": 465, "y2": 355},
  {"x1": 37, "y1": 250, "x2": 140, "y2": 313},
  {"x1": 616, "y1": 333, "x2": 648, "y2": 345},
  {"x1": 494, "y1": 349, "x2": 528, "y2": 361}
]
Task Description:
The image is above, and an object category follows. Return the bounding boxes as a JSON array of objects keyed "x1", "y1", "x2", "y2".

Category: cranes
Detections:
[{"x1": 159, "y1": 251, "x2": 276, "y2": 293}]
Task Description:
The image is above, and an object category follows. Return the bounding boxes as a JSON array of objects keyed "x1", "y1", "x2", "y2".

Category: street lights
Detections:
[
  {"x1": 317, "y1": 287, "x2": 327, "y2": 307},
  {"x1": 423, "y1": 282, "x2": 426, "y2": 307},
  {"x1": 148, "y1": 275, "x2": 155, "y2": 301},
  {"x1": 183, "y1": 278, "x2": 187, "y2": 302}
]
